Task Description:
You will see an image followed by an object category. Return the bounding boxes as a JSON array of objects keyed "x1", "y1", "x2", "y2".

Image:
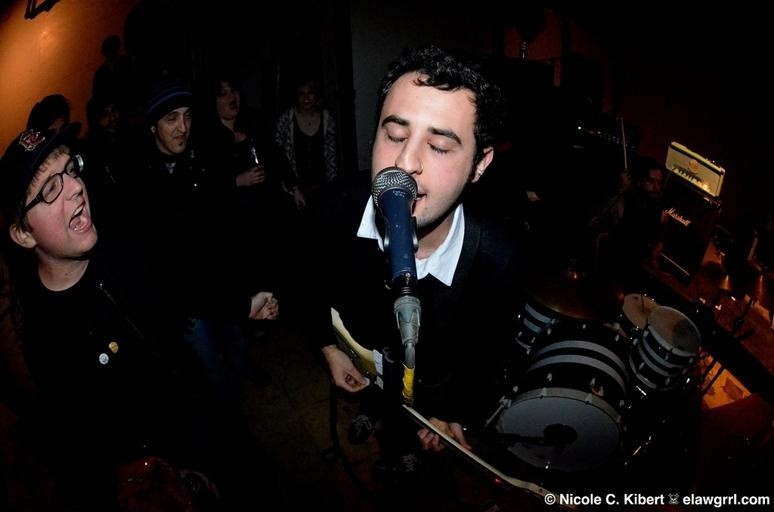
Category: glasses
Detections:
[{"x1": 24, "y1": 154, "x2": 84, "y2": 212}]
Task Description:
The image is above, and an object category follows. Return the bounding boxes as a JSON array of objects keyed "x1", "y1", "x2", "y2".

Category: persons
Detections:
[
  {"x1": 595, "y1": 154, "x2": 676, "y2": 294},
  {"x1": 307, "y1": 45, "x2": 532, "y2": 512},
  {"x1": 0, "y1": 72, "x2": 344, "y2": 512}
]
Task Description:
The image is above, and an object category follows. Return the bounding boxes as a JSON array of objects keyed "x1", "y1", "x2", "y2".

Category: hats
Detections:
[
  {"x1": 1, "y1": 122, "x2": 81, "y2": 225},
  {"x1": 148, "y1": 84, "x2": 192, "y2": 125}
]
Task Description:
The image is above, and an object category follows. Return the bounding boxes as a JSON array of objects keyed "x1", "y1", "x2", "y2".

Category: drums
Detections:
[
  {"x1": 495, "y1": 311, "x2": 631, "y2": 474},
  {"x1": 509, "y1": 276, "x2": 592, "y2": 352},
  {"x1": 625, "y1": 305, "x2": 702, "y2": 395},
  {"x1": 616, "y1": 290, "x2": 662, "y2": 341}
]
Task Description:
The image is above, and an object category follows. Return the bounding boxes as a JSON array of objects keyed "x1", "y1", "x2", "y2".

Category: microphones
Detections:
[{"x1": 370, "y1": 166, "x2": 423, "y2": 344}]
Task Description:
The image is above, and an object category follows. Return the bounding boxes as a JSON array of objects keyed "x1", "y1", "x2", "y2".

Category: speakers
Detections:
[{"x1": 651, "y1": 171, "x2": 725, "y2": 278}]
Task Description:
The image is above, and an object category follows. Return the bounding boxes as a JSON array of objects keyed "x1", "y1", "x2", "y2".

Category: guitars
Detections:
[{"x1": 327, "y1": 306, "x2": 579, "y2": 512}]
